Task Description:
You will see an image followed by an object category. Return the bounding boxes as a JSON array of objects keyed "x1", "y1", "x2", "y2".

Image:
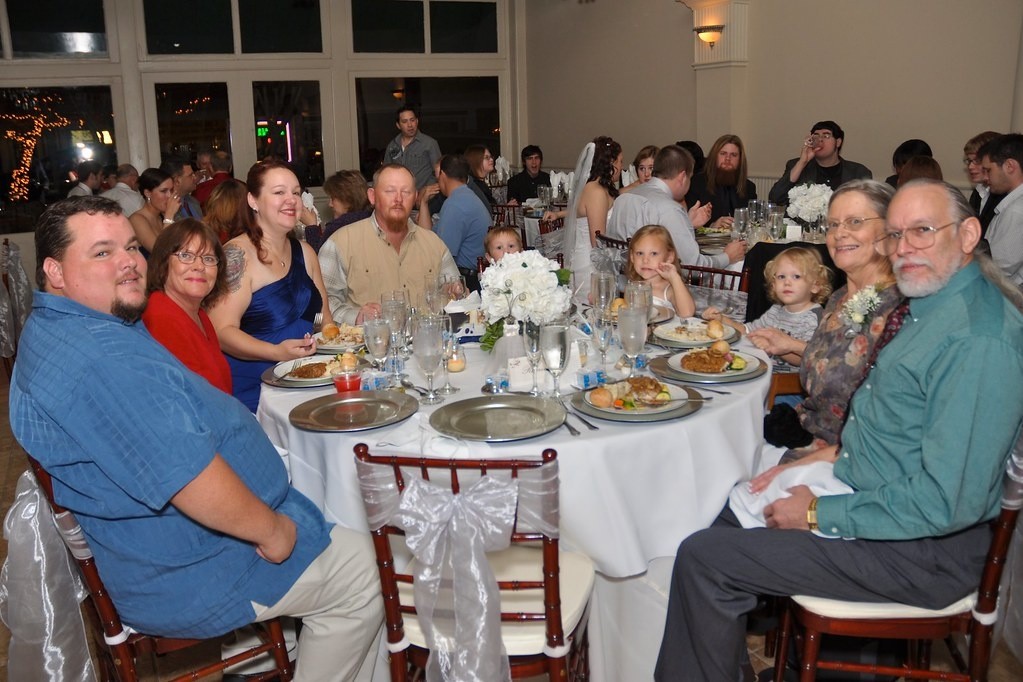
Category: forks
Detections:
[
  {"x1": 272, "y1": 360, "x2": 302, "y2": 384},
  {"x1": 680, "y1": 318, "x2": 688, "y2": 325},
  {"x1": 308, "y1": 312, "x2": 323, "y2": 339},
  {"x1": 555, "y1": 399, "x2": 599, "y2": 431}
]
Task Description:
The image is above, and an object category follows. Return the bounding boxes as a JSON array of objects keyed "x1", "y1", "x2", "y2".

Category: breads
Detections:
[
  {"x1": 321, "y1": 323, "x2": 340, "y2": 340},
  {"x1": 340, "y1": 352, "x2": 357, "y2": 371},
  {"x1": 589, "y1": 387, "x2": 613, "y2": 408},
  {"x1": 711, "y1": 341, "x2": 729, "y2": 355},
  {"x1": 611, "y1": 297, "x2": 625, "y2": 311}
]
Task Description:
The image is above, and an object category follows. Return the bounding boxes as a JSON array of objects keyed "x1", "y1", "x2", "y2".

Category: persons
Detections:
[
  {"x1": 7, "y1": 195, "x2": 386, "y2": 682},
  {"x1": 656, "y1": 177, "x2": 1023, "y2": 682},
  {"x1": 7, "y1": 106, "x2": 1023, "y2": 682}
]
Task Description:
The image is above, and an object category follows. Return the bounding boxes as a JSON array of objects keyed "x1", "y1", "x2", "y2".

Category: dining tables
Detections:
[
  {"x1": 253, "y1": 315, "x2": 772, "y2": 682},
  {"x1": 696, "y1": 234, "x2": 827, "y2": 291}
]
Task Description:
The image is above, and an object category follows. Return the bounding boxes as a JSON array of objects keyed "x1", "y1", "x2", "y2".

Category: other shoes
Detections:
[{"x1": 223, "y1": 672, "x2": 283, "y2": 682}]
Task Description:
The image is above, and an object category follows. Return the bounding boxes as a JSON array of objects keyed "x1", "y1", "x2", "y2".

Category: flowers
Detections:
[
  {"x1": 478, "y1": 249, "x2": 576, "y2": 355},
  {"x1": 786, "y1": 184, "x2": 834, "y2": 223}
]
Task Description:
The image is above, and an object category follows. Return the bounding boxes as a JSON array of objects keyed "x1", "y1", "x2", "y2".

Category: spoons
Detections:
[
  {"x1": 713, "y1": 307, "x2": 734, "y2": 315},
  {"x1": 401, "y1": 379, "x2": 425, "y2": 395}
]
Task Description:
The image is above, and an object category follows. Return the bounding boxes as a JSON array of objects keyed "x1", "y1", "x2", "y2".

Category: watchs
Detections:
[{"x1": 807, "y1": 498, "x2": 819, "y2": 530}]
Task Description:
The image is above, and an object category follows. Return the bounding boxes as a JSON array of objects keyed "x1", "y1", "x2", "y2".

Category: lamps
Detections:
[{"x1": 693, "y1": 25, "x2": 724, "y2": 48}]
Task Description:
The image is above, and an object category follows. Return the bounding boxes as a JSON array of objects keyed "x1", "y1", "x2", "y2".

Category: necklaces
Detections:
[
  {"x1": 259, "y1": 240, "x2": 287, "y2": 268},
  {"x1": 830, "y1": 316, "x2": 838, "y2": 328}
]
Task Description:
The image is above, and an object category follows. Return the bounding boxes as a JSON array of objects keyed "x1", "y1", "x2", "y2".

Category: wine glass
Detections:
[
  {"x1": 364, "y1": 272, "x2": 466, "y2": 406},
  {"x1": 590, "y1": 272, "x2": 654, "y2": 383},
  {"x1": 536, "y1": 183, "x2": 564, "y2": 210},
  {"x1": 523, "y1": 316, "x2": 571, "y2": 400}
]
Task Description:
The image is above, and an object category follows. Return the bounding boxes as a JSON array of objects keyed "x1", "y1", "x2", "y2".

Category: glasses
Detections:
[
  {"x1": 820, "y1": 217, "x2": 882, "y2": 234},
  {"x1": 484, "y1": 155, "x2": 494, "y2": 160},
  {"x1": 873, "y1": 219, "x2": 966, "y2": 257},
  {"x1": 171, "y1": 251, "x2": 218, "y2": 266},
  {"x1": 814, "y1": 134, "x2": 834, "y2": 140},
  {"x1": 963, "y1": 158, "x2": 983, "y2": 165}
]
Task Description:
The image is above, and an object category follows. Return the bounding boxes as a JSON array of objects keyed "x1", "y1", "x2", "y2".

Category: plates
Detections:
[
  {"x1": 583, "y1": 302, "x2": 675, "y2": 325},
  {"x1": 311, "y1": 327, "x2": 368, "y2": 354},
  {"x1": 570, "y1": 378, "x2": 703, "y2": 423},
  {"x1": 288, "y1": 388, "x2": 420, "y2": 433},
  {"x1": 647, "y1": 315, "x2": 740, "y2": 348},
  {"x1": 260, "y1": 355, "x2": 373, "y2": 388},
  {"x1": 428, "y1": 395, "x2": 567, "y2": 443},
  {"x1": 649, "y1": 346, "x2": 769, "y2": 385}
]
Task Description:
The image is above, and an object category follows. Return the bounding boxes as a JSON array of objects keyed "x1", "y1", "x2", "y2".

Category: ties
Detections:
[{"x1": 183, "y1": 199, "x2": 194, "y2": 218}]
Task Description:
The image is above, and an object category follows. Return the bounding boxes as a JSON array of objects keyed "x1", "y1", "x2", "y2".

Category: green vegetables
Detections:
[{"x1": 695, "y1": 227, "x2": 722, "y2": 236}]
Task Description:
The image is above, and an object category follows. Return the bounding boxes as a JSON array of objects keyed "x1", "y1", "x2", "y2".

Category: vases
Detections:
[{"x1": 487, "y1": 322, "x2": 532, "y2": 376}]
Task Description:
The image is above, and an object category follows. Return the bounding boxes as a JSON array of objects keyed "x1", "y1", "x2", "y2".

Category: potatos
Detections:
[{"x1": 707, "y1": 319, "x2": 724, "y2": 338}]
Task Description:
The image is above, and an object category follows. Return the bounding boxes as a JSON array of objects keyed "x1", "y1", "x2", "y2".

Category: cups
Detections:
[
  {"x1": 809, "y1": 207, "x2": 827, "y2": 242},
  {"x1": 803, "y1": 136, "x2": 823, "y2": 149},
  {"x1": 490, "y1": 170, "x2": 511, "y2": 186},
  {"x1": 730, "y1": 199, "x2": 784, "y2": 243}
]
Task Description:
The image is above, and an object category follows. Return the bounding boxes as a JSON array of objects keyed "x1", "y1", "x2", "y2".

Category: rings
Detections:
[{"x1": 173, "y1": 195, "x2": 178, "y2": 199}]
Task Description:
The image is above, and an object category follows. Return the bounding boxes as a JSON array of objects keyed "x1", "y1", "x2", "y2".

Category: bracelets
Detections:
[{"x1": 163, "y1": 219, "x2": 175, "y2": 224}]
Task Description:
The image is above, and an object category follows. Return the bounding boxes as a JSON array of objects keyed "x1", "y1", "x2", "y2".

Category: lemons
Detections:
[
  {"x1": 731, "y1": 358, "x2": 746, "y2": 370},
  {"x1": 619, "y1": 397, "x2": 634, "y2": 408},
  {"x1": 654, "y1": 392, "x2": 671, "y2": 401}
]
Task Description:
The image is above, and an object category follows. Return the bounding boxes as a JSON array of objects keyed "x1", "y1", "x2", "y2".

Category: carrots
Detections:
[{"x1": 615, "y1": 400, "x2": 624, "y2": 406}]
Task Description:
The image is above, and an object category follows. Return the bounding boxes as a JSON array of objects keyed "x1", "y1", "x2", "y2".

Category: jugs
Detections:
[{"x1": 447, "y1": 346, "x2": 465, "y2": 373}]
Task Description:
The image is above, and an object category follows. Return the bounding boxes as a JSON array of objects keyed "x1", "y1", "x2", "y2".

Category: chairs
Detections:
[{"x1": 0, "y1": 201, "x2": 1023, "y2": 682}]
